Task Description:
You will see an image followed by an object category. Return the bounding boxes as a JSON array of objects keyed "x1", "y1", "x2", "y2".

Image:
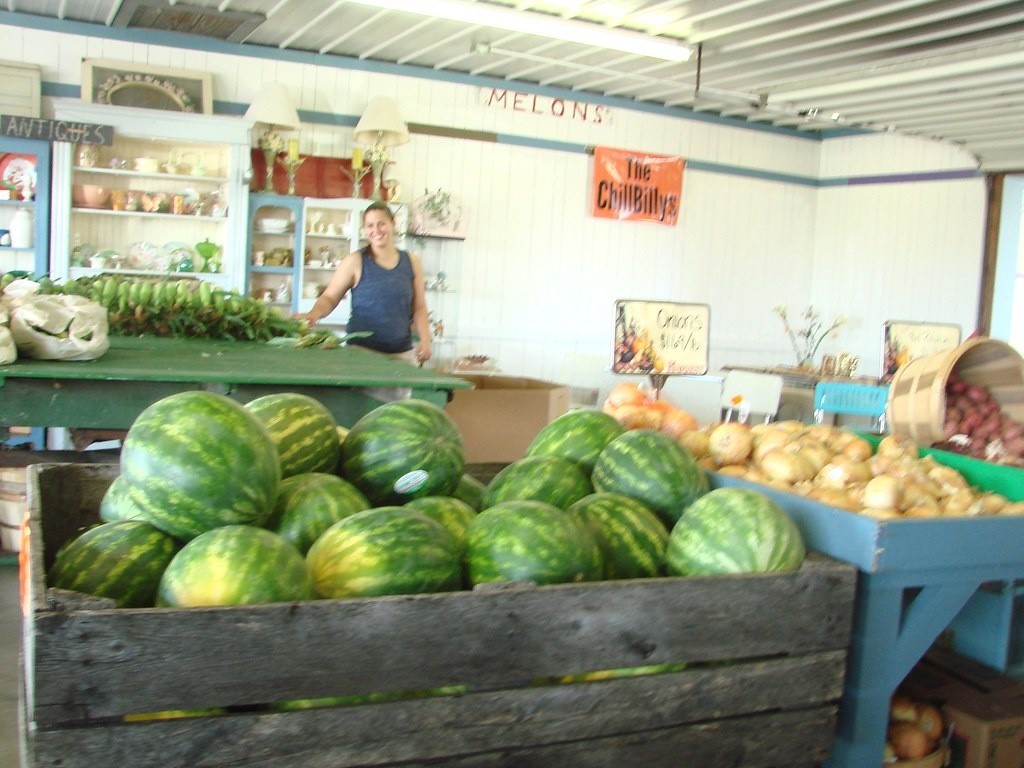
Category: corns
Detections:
[{"x1": 0, "y1": 275, "x2": 315, "y2": 343}]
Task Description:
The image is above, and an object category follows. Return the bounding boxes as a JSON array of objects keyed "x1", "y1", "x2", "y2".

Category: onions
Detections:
[
  {"x1": 600, "y1": 383, "x2": 1023, "y2": 520},
  {"x1": 885, "y1": 696, "x2": 943, "y2": 766}
]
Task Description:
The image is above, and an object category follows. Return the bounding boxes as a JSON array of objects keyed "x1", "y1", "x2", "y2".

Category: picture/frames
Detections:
[{"x1": 81, "y1": 56, "x2": 213, "y2": 115}]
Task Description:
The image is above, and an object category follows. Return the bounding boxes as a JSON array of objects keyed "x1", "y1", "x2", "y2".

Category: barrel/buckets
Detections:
[{"x1": 885, "y1": 335, "x2": 1024, "y2": 452}]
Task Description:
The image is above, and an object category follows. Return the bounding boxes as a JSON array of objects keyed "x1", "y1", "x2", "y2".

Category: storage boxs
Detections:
[{"x1": 18, "y1": 376, "x2": 1023, "y2": 768}]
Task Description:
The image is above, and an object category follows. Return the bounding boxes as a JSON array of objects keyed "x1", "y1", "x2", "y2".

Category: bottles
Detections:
[
  {"x1": 0, "y1": 230, "x2": 11, "y2": 246},
  {"x1": 254, "y1": 251, "x2": 264, "y2": 266}
]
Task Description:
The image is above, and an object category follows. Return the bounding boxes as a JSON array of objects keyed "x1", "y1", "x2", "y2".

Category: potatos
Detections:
[{"x1": 940, "y1": 377, "x2": 1024, "y2": 468}]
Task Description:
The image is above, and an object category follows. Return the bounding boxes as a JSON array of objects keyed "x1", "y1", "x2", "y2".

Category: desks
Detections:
[
  {"x1": 0, "y1": 294, "x2": 472, "y2": 435},
  {"x1": 719, "y1": 367, "x2": 879, "y2": 429}
]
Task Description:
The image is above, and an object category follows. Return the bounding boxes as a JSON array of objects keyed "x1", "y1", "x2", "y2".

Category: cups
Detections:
[{"x1": 820, "y1": 355, "x2": 837, "y2": 375}]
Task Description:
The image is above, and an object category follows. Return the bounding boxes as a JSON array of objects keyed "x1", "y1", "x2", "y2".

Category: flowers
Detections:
[{"x1": 773, "y1": 304, "x2": 845, "y2": 367}]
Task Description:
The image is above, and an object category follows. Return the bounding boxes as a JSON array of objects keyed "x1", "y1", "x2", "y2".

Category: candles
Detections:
[
  {"x1": 288, "y1": 139, "x2": 299, "y2": 161},
  {"x1": 352, "y1": 147, "x2": 363, "y2": 169}
]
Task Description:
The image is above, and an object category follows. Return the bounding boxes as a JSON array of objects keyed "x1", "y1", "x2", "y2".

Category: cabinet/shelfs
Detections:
[{"x1": 0, "y1": 136, "x2": 467, "y2": 343}]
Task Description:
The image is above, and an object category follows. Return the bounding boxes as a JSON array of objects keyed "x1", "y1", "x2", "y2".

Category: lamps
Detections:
[
  {"x1": 353, "y1": 94, "x2": 410, "y2": 201},
  {"x1": 356, "y1": 1, "x2": 695, "y2": 64},
  {"x1": 241, "y1": 93, "x2": 303, "y2": 194}
]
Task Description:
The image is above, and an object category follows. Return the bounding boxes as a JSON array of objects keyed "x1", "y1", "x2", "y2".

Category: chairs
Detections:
[
  {"x1": 718, "y1": 371, "x2": 783, "y2": 427},
  {"x1": 812, "y1": 383, "x2": 891, "y2": 437}
]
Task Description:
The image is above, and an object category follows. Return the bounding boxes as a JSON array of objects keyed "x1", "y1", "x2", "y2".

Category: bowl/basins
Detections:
[
  {"x1": 133, "y1": 155, "x2": 158, "y2": 172},
  {"x1": 90, "y1": 257, "x2": 106, "y2": 267},
  {"x1": 72, "y1": 184, "x2": 111, "y2": 208}
]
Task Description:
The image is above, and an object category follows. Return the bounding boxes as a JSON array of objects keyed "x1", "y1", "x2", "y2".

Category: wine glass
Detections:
[{"x1": 308, "y1": 211, "x2": 322, "y2": 233}]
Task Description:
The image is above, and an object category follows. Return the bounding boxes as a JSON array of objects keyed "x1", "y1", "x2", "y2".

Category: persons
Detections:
[{"x1": 287, "y1": 201, "x2": 431, "y2": 402}]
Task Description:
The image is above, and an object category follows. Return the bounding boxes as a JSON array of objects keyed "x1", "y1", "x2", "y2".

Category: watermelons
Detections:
[{"x1": 50, "y1": 391, "x2": 810, "y2": 724}]
